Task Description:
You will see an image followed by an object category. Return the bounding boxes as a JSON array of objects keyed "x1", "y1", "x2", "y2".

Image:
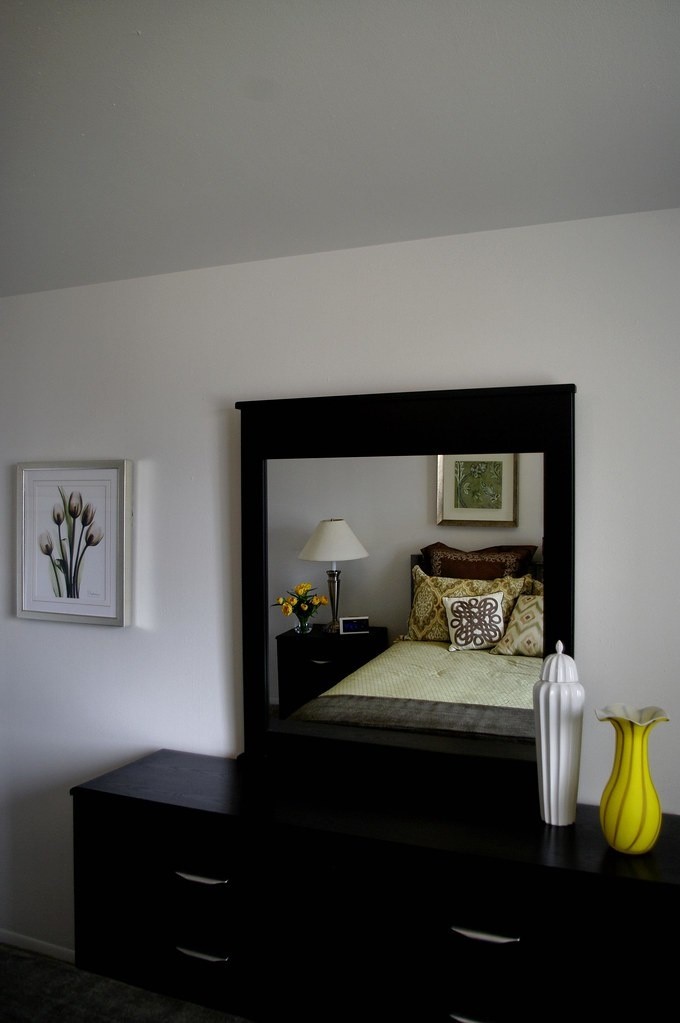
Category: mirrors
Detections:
[{"x1": 232, "y1": 384, "x2": 576, "y2": 810}]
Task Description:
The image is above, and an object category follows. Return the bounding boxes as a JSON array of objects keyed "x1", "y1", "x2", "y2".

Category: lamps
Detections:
[{"x1": 297, "y1": 515, "x2": 369, "y2": 635}]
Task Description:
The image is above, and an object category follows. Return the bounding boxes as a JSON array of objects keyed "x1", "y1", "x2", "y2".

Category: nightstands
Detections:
[{"x1": 276, "y1": 622, "x2": 389, "y2": 719}]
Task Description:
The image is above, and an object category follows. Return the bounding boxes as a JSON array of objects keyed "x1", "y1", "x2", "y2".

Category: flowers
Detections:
[{"x1": 270, "y1": 582, "x2": 329, "y2": 634}]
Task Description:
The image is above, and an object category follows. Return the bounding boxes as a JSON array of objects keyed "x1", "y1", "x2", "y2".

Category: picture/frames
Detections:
[
  {"x1": 436, "y1": 454, "x2": 520, "y2": 528},
  {"x1": 12, "y1": 457, "x2": 130, "y2": 628}
]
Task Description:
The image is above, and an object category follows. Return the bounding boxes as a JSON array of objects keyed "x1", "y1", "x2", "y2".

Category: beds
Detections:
[{"x1": 291, "y1": 555, "x2": 547, "y2": 763}]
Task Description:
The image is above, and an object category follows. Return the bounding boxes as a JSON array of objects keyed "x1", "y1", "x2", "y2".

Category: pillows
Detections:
[{"x1": 404, "y1": 542, "x2": 544, "y2": 657}]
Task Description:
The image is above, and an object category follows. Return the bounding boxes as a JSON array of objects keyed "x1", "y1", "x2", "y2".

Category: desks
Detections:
[{"x1": 69, "y1": 750, "x2": 679, "y2": 1023}]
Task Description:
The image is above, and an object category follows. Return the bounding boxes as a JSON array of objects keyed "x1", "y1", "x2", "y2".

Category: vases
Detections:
[
  {"x1": 294, "y1": 611, "x2": 312, "y2": 633},
  {"x1": 594, "y1": 704, "x2": 670, "y2": 853}
]
